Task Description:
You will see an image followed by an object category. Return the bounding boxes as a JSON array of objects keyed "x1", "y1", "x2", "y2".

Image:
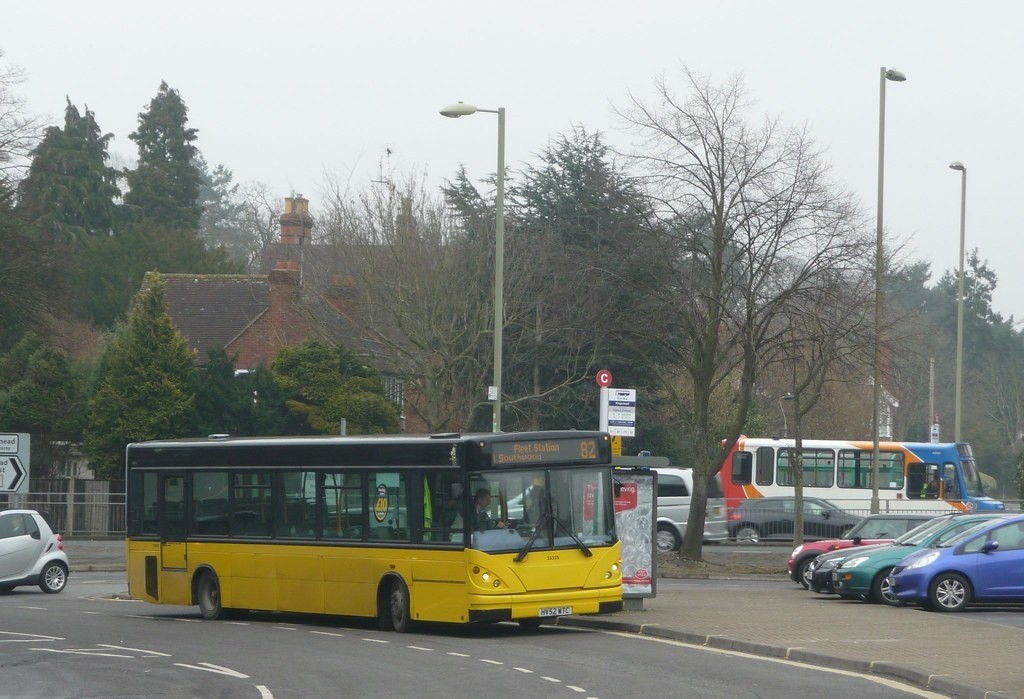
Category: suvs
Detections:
[
  {"x1": 485, "y1": 466, "x2": 729, "y2": 552},
  {"x1": 727, "y1": 496, "x2": 864, "y2": 548}
]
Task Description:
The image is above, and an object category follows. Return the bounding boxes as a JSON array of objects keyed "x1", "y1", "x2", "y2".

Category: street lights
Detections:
[
  {"x1": 948, "y1": 162, "x2": 967, "y2": 444},
  {"x1": 867, "y1": 65, "x2": 907, "y2": 517},
  {"x1": 438, "y1": 100, "x2": 506, "y2": 522}
]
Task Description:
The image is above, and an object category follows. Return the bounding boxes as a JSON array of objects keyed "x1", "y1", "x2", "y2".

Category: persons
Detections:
[
  {"x1": 448, "y1": 487, "x2": 510, "y2": 544},
  {"x1": 926, "y1": 480, "x2": 941, "y2": 499}
]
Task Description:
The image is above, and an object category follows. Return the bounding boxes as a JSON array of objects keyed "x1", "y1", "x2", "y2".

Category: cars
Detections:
[
  {"x1": 787, "y1": 510, "x2": 1024, "y2": 607},
  {"x1": 0, "y1": 509, "x2": 69, "y2": 594},
  {"x1": 888, "y1": 513, "x2": 1024, "y2": 612}
]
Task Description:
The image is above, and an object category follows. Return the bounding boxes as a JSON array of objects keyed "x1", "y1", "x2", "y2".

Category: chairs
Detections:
[
  {"x1": 152, "y1": 495, "x2": 397, "y2": 539},
  {"x1": 1018, "y1": 521, "x2": 1024, "y2": 548},
  {"x1": 496, "y1": 518, "x2": 525, "y2": 533}
]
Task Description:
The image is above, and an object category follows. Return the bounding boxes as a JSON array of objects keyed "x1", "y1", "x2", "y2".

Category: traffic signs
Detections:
[{"x1": 0, "y1": 454, "x2": 28, "y2": 493}]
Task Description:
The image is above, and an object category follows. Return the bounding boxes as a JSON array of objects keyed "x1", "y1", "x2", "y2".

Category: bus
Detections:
[
  {"x1": 125, "y1": 429, "x2": 626, "y2": 634},
  {"x1": 720, "y1": 435, "x2": 1007, "y2": 543}
]
